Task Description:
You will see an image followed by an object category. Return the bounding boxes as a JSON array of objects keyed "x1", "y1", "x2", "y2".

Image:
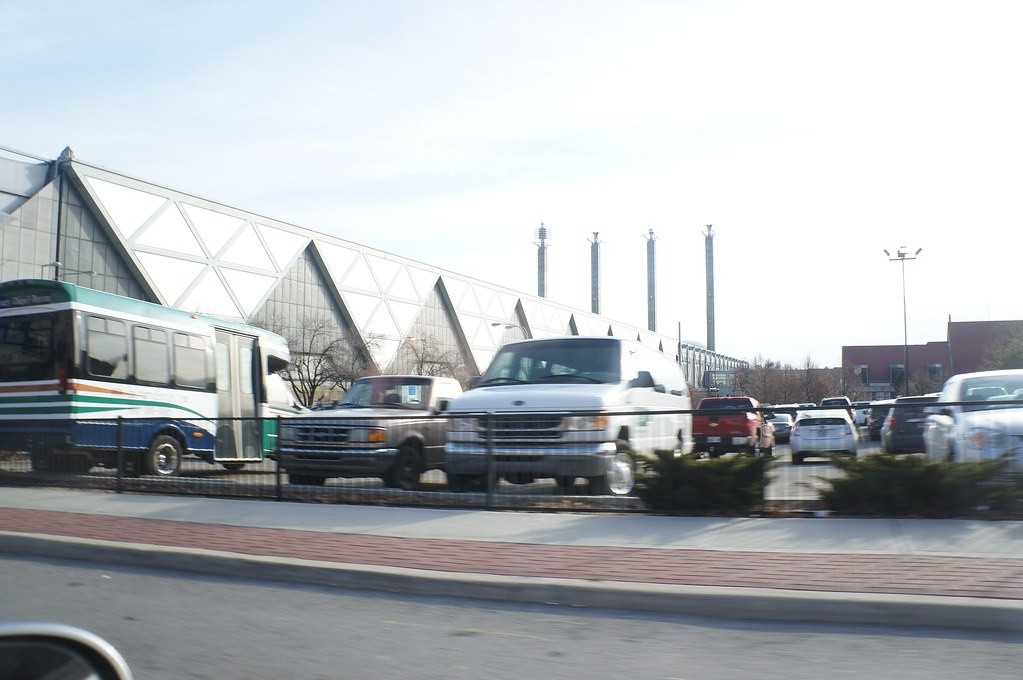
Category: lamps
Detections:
[
  {"x1": 72, "y1": 271, "x2": 97, "y2": 276},
  {"x1": 43, "y1": 262, "x2": 61, "y2": 266}
]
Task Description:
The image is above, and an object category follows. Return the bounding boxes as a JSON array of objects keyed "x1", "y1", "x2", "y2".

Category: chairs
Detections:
[
  {"x1": 385, "y1": 394, "x2": 400, "y2": 403},
  {"x1": 528, "y1": 368, "x2": 552, "y2": 382}
]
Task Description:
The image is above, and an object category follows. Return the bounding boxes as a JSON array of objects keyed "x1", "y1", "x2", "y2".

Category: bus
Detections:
[{"x1": 0, "y1": 280, "x2": 293, "y2": 477}]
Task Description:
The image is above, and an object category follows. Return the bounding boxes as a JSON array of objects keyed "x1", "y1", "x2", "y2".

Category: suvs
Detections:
[
  {"x1": 820, "y1": 396, "x2": 856, "y2": 423},
  {"x1": 446, "y1": 336, "x2": 692, "y2": 497},
  {"x1": 922, "y1": 368, "x2": 1023, "y2": 479},
  {"x1": 880, "y1": 396, "x2": 939, "y2": 455},
  {"x1": 869, "y1": 399, "x2": 897, "y2": 441},
  {"x1": 693, "y1": 397, "x2": 776, "y2": 458},
  {"x1": 276, "y1": 374, "x2": 461, "y2": 490}
]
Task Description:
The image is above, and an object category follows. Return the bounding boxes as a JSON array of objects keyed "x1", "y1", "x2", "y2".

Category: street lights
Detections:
[{"x1": 884, "y1": 247, "x2": 923, "y2": 396}]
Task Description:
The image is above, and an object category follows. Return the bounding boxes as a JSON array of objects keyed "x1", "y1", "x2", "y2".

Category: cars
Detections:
[
  {"x1": 851, "y1": 401, "x2": 871, "y2": 426},
  {"x1": 763, "y1": 402, "x2": 816, "y2": 440},
  {"x1": 789, "y1": 411, "x2": 859, "y2": 464}
]
